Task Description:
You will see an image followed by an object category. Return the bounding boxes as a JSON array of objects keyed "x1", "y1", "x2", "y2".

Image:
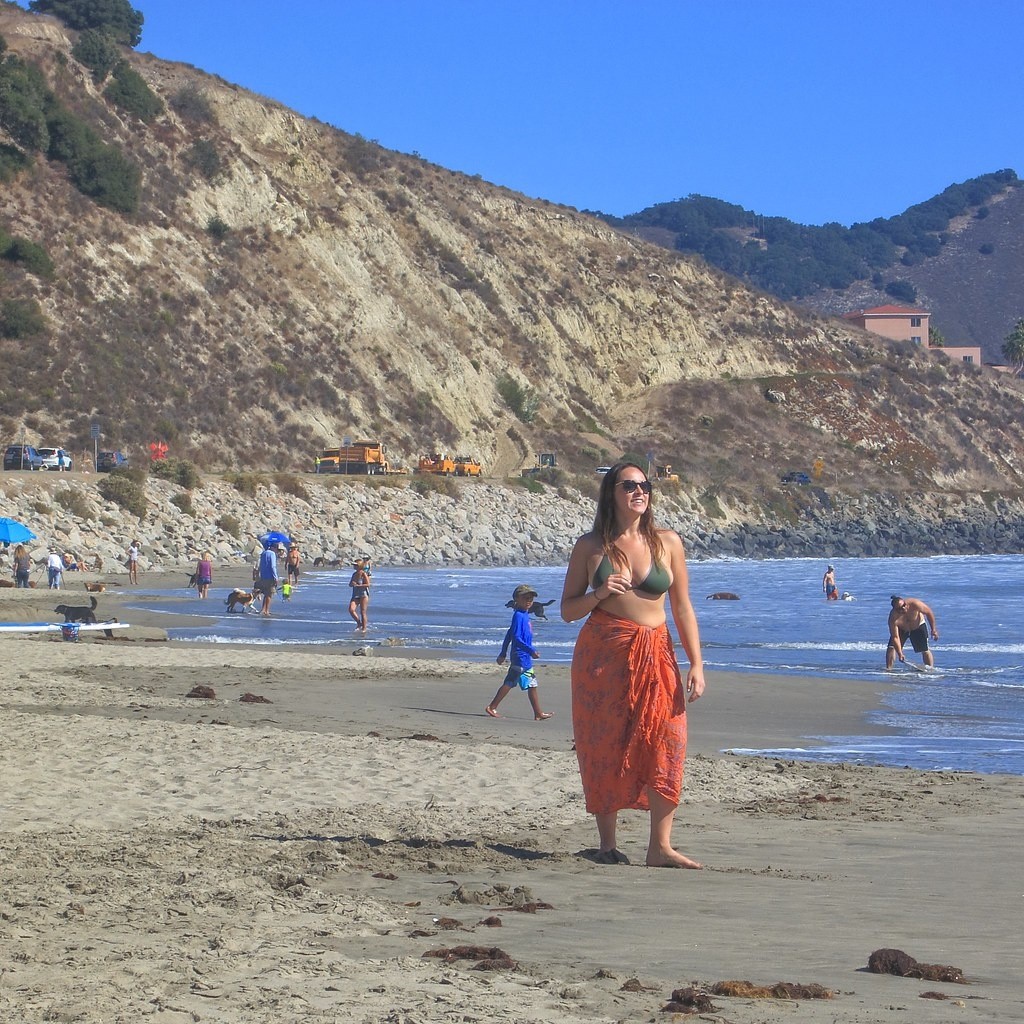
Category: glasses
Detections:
[
  {"x1": 614, "y1": 479, "x2": 652, "y2": 493},
  {"x1": 897, "y1": 603, "x2": 906, "y2": 611}
]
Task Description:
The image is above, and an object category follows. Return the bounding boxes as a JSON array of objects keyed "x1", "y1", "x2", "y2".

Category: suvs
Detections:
[
  {"x1": 36, "y1": 447, "x2": 73, "y2": 471},
  {"x1": 3, "y1": 444, "x2": 44, "y2": 471},
  {"x1": 94, "y1": 452, "x2": 129, "y2": 473}
]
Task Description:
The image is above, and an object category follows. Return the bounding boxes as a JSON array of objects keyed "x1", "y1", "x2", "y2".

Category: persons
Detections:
[
  {"x1": 196, "y1": 552, "x2": 213, "y2": 599},
  {"x1": 362, "y1": 558, "x2": 371, "y2": 581},
  {"x1": 278, "y1": 579, "x2": 293, "y2": 602},
  {"x1": 47, "y1": 547, "x2": 63, "y2": 589},
  {"x1": 13, "y1": 545, "x2": 31, "y2": 589},
  {"x1": 57, "y1": 447, "x2": 66, "y2": 472},
  {"x1": 823, "y1": 564, "x2": 838, "y2": 600},
  {"x1": 886, "y1": 595, "x2": 939, "y2": 670},
  {"x1": 251, "y1": 541, "x2": 279, "y2": 616},
  {"x1": 285, "y1": 542, "x2": 300, "y2": 586},
  {"x1": 485, "y1": 585, "x2": 554, "y2": 721},
  {"x1": 62, "y1": 552, "x2": 89, "y2": 572},
  {"x1": 125, "y1": 539, "x2": 142, "y2": 585},
  {"x1": 348, "y1": 559, "x2": 370, "y2": 633}
]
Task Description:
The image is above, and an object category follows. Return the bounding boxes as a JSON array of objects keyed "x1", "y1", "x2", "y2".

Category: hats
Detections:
[
  {"x1": 289, "y1": 542, "x2": 298, "y2": 548},
  {"x1": 512, "y1": 584, "x2": 538, "y2": 598},
  {"x1": 828, "y1": 564, "x2": 835, "y2": 570},
  {"x1": 363, "y1": 555, "x2": 370, "y2": 560},
  {"x1": 49, "y1": 548, "x2": 57, "y2": 554}
]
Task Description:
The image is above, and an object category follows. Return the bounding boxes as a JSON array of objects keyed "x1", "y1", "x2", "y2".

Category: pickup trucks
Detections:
[
  {"x1": 780, "y1": 471, "x2": 812, "y2": 487},
  {"x1": 453, "y1": 455, "x2": 482, "y2": 478},
  {"x1": 418, "y1": 450, "x2": 459, "y2": 476}
]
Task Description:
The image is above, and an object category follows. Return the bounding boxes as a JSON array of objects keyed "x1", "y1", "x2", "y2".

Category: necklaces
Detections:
[{"x1": 560, "y1": 461, "x2": 705, "y2": 868}]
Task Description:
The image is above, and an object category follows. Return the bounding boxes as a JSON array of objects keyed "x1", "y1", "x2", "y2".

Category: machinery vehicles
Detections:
[
  {"x1": 651, "y1": 464, "x2": 679, "y2": 483},
  {"x1": 526, "y1": 453, "x2": 559, "y2": 479}
]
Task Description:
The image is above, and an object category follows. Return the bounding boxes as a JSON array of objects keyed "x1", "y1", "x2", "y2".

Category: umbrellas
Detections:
[
  {"x1": 0, "y1": 517, "x2": 37, "y2": 544},
  {"x1": 257, "y1": 530, "x2": 292, "y2": 548}
]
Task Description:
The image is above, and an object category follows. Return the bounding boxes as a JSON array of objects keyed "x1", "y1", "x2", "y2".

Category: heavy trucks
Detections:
[
  {"x1": 318, "y1": 448, "x2": 342, "y2": 474},
  {"x1": 339, "y1": 440, "x2": 388, "y2": 475}
]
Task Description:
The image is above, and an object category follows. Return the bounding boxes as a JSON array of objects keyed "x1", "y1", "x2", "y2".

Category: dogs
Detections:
[
  {"x1": 53, "y1": 596, "x2": 98, "y2": 624},
  {"x1": 705, "y1": 593, "x2": 742, "y2": 601},
  {"x1": 83, "y1": 581, "x2": 107, "y2": 593},
  {"x1": 224, "y1": 589, "x2": 262, "y2": 614},
  {"x1": 841, "y1": 592, "x2": 856, "y2": 602},
  {"x1": 1, "y1": 579, "x2": 37, "y2": 588},
  {"x1": 185, "y1": 572, "x2": 200, "y2": 589},
  {"x1": 314, "y1": 553, "x2": 344, "y2": 568},
  {"x1": 505, "y1": 600, "x2": 557, "y2": 621}
]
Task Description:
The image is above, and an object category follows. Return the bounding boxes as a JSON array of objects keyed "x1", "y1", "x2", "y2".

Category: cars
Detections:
[{"x1": 594, "y1": 466, "x2": 612, "y2": 475}]
